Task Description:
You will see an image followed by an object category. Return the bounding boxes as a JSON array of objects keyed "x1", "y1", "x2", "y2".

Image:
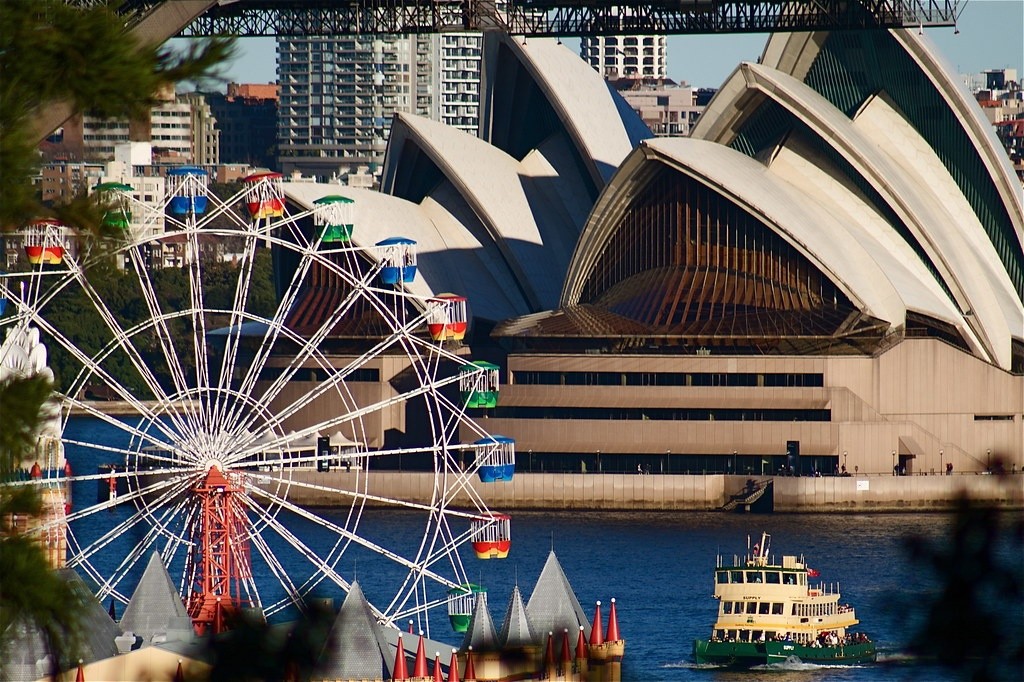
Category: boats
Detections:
[{"x1": 693, "y1": 531, "x2": 877, "y2": 670}]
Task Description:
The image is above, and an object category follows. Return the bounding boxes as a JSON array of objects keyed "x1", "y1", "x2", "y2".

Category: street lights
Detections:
[
  {"x1": 843, "y1": 450, "x2": 847, "y2": 474},
  {"x1": 786, "y1": 451, "x2": 790, "y2": 475},
  {"x1": 398, "y1": 447, "x2": 402, "y2": 473},
  {"x1": 892, "y1": 451, "x2": 895, "y2": 476},
  {"x1": 528, "y1": 449, "x2": 532, "y2": 472},
  {"x1": 734, "y1": 450, "x2": 738, "y2": 474},
  {"x1": 940, "y1": 450, "x2": 943, "y2": 475},
  {"x1": 987, "y1": 449, "x2": 990, "y2": 469},
  {"x1": 667, "y1": 449, "x2": 670, "y2": 474},
  {"x1": 597, "y1": 450, "x2": 600, "y2": 471}
]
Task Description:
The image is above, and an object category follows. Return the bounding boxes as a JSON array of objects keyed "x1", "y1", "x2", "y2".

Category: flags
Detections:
[{"x1": 807, "y1": 568, "x2": 821, "y2": 578}]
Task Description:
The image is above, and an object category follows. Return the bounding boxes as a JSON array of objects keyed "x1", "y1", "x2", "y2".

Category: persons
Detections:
[
  {"x1": 896, "y1": 464, "x2": 899, "y2": 476},
  {"x1": 380, "y1": 257, "x2": 390, "y2": 267},
  {"x1": 835, "y1": 464, "x2": 838, "y2": 476},
  {"x1": 798, "y1": 631, "x2": 869, "y2": 649},
  {"x1": 335, "y1": 458, "x2": 351, "y2": 466},
  {"x1": 714, "y1": 634, "x2": 761, "y2": 644},
  {"x1": 947, "y1": 463, "x2": 953, "y2": 475},
  {"x1": 638, "y1": 464, "x2": 649, "y2": 475},
  {"x1": 769, "y1": 632, "x2": 791, "y2": 642},
  {"x1": 842, "y1": 464, "x2": 845, "y2": 473},
  {"x1": 1011, "y1": 464, "x2": 1017, "y2": 475}
]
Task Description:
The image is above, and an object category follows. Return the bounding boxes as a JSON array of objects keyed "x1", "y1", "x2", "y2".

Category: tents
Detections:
[{"x1": 174, "y1": 428, "x2": 363, "y2": 466}]
